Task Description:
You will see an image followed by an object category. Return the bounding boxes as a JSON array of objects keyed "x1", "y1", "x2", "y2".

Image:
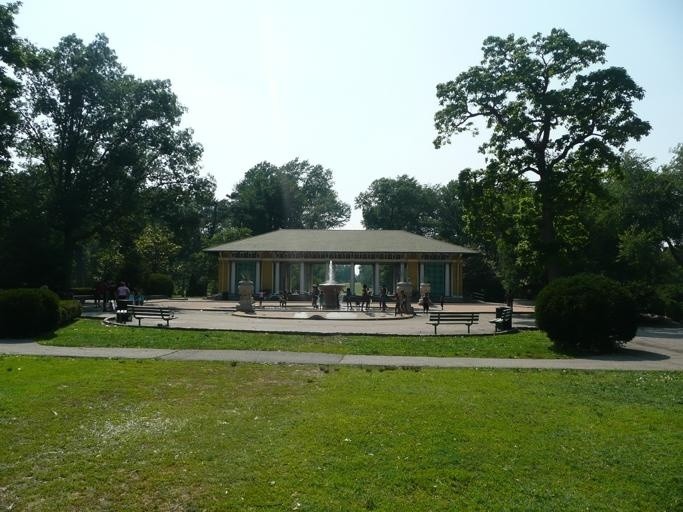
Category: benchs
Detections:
[
  {"x1": 72, "y1": 294, "x2": 102, "y2": 304},
  {"x1": 425, "y1": 307, "x2": 512, "y2": 335},
  {"x1": 343, "y1": 296, "x2": 394, "y2": 310},
  {"x1": 128, "y1": 306, "x2": 179, "y2": 326}
]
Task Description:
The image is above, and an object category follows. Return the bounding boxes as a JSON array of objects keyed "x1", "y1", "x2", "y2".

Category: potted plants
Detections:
[{"x1": 236, "y1": 270, "x2": 256, "y2": 314}]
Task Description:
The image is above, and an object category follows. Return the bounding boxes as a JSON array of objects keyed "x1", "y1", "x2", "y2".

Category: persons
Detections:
[
  {"x1": 39, "y1": 280, "x2": 48, "y2": 290},
  {"x1": 279, "y1": 290, "x2": 289, "y2": 308},
  {"x1": 440, "y1": 291, "x2": 444, "y2": 309},
  {"x1": 311, "y1": 284, "x2": 434, "y2": 318},
  {"x1": 92, "y1": 278, "x2": 145, "y2": 308},
  {"x1": 258, "y1": 289, "x2": 264, "y2": 306}
]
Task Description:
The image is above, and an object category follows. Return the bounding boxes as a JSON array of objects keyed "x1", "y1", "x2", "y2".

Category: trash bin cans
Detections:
[{"x1": 117, "y1": 300, "x2": 133, "y2": 321}]
